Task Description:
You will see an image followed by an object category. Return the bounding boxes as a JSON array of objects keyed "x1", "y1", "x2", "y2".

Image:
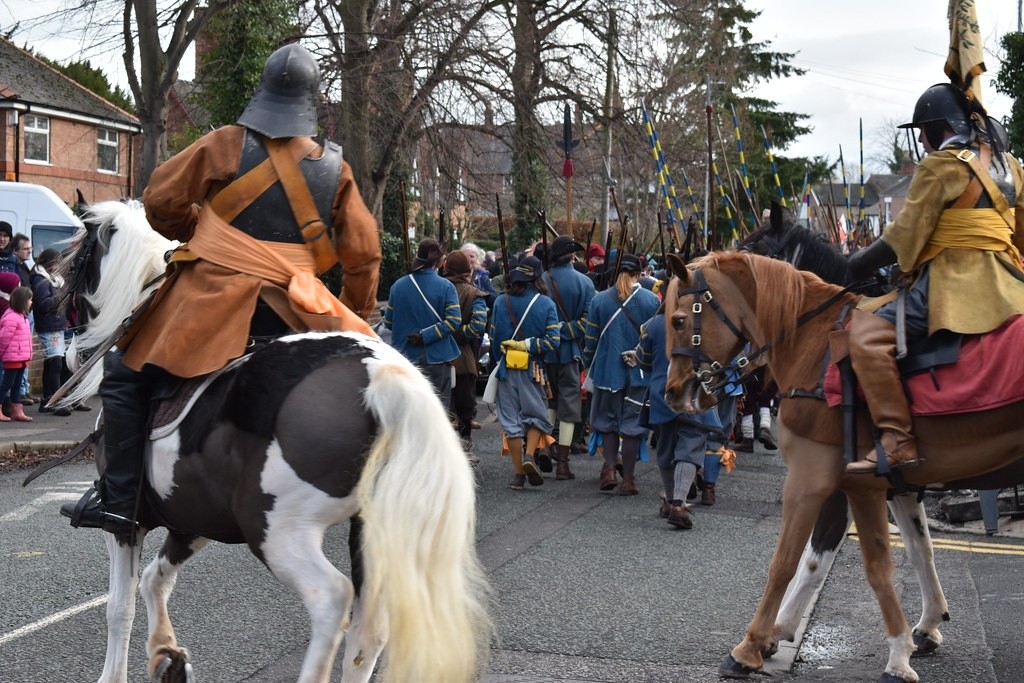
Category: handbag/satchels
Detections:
[
  {"x1": 450, "y1": 366, "x2": 456, "y2": 389},
  {"x1": 506, "y1": 349, "x2": 530, "y2": 370},
  {"x1": 580, "y1": 373, "x2": 595, "y2": 393},
  {"x1": 482, "y1": 361, "x2": 502, "y2": 403}
]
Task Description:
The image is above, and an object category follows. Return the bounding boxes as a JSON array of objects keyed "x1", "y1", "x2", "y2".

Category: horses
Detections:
[
  {"x1": 34, "y1": 188, "x2": 501, "y2": 683},
  {"x1": 664, "y1": 251, "x2": 1024, "y2": 683},
  {"x1": 726, "y1": 188, "x2": 1024, "y2": 657}
]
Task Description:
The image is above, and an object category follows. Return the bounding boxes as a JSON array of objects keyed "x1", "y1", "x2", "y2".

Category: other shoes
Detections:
[
  {"x1": 450, "y1": 408, "x2": 780, "y2": 529},
  {"x1": 26, "y1": 393, "x2": 41, "y2": 403},
  {"x1": 39, "y1": 400, "x2": 54, "y2": 412},
  {"x1": 56, "y1": 408, "x2": 71, "y2": 416},
  {"x1": 72, "y1": 401, "x2": 92, "y2": 411},
  {"x1": 19, "y1": 394, "x2": 34, "y2": 405}
]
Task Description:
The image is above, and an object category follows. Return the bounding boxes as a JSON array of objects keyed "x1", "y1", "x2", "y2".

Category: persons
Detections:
[
  {"x1": 384, "y1": 235, "x2": 777, "y2": 529},
  {"x1": 60, "y1": 41, "x2": 382, "y2": 533},
  {"x1": 0, "y1": 221, "x2": 93, "y2": 421},
  {"x1": 843, "y1": 83, "x2": 1023, "y2": 474}
]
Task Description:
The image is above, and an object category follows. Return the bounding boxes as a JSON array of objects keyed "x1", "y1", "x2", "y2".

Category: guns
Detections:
[
  {"x1": 537, "y1": 209, "x2": 548, "y2": 268},
  {"x1": 581, "y1": 212, "x2": 701, "y2": 290},
  {"x1": 495, "y1": 192, "x2": 510, "y2": 290},
  {"x1": 439, "y1": 199, "x2": 444, "y2": 277}
]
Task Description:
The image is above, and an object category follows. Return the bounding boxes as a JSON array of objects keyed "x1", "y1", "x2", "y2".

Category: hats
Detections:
[
  {"x1": 0, "y1": 221, "x2": 12, "y2": 241},
  {"x1": 0, "y1": 272, "x2": 21, "y2": 294},
  {"x1": 408, "y1": 234, "x2": 706, "y2": 282}
]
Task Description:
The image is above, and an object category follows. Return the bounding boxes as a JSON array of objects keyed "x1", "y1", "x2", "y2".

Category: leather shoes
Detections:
[{"x1": 846, "y1": 430, "x2": 922, "y2": 474}]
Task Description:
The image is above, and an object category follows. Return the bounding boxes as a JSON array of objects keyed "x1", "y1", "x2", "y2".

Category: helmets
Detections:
[
  {"x1": 894, "y1": 83, "x2": 976, "y2": 136},
  {"x1": 234, "y1": 41, "x2": 320, "y2": 140}
]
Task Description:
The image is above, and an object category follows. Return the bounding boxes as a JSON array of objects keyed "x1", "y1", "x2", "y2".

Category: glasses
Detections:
[{"x1": 17, "y1": 246, "x2": 33, "y2": 252}]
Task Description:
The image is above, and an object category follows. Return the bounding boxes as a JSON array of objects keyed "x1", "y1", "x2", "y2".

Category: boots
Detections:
[
  {"x1": 10, "y1": 403, "x2": 33, "y2": 422},
  {"x1": 58, "y1": 355, "x2": 158, "y2": 533},
  {"x1": 0, "y1": 404, "x2": 12, "y2": 421}
]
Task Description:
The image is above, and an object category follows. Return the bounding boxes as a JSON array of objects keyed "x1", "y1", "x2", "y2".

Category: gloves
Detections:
[
  {"x1": 499, "y1": 338, "x2": 527, "y2": 355},
  {"x1": 621, "y1": 350, "x2": 638, "y2": 367},
  {"x1": 842, "y1": 239, "x2": 898, "y2": 284}
]
